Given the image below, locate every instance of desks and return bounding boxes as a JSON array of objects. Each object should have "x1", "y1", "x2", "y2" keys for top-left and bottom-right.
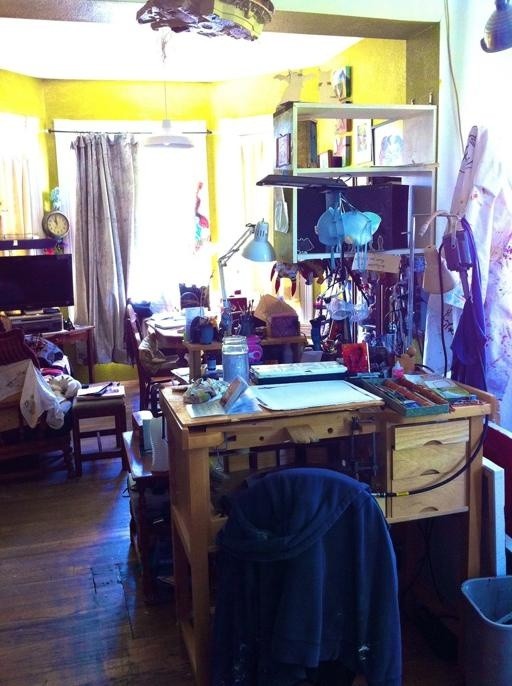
[
  {"x1": 0, "y1": 325, "x2": 94, "y2": 386},
  {"x1": 159, "y1": 371, "x2": 497, "y2": 686}
]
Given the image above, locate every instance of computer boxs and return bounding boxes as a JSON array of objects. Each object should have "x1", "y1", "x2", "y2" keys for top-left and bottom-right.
[{"x1": 8, "y1": 312, "x2": 64, "y2": 335}]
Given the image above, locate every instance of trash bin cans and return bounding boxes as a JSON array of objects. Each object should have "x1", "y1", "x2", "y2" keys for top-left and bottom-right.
[{"x1": 460, "y1": 576, "x2": 511, "y2": 686}]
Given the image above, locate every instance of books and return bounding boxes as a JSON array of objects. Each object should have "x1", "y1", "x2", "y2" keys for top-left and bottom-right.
[{"x1": 184, "y1": 375, "x2": 263, "y2": 417}]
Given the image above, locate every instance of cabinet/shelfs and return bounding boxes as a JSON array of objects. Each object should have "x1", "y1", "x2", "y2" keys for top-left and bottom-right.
[{"x1": 272, "y1": 105, "x2": 435, "y2": 263}]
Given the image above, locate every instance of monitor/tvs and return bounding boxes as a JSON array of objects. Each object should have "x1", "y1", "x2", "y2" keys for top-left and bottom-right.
[{"x1": 0, "y1": 252, "x2": 73, "y2": 313}]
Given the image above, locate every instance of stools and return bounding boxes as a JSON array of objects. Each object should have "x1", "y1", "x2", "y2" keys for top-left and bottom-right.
[{"x1": 72, "y1": 383, "x2": 129, "y2": 473}]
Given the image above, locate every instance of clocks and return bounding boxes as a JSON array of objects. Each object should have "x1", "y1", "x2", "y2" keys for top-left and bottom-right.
[{"x1": 43, "y1": 211, "x2": 70, "y2": 239}]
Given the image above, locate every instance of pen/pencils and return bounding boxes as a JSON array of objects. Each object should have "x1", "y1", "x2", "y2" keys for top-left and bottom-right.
[{"x1": 236, "y1": 299, "x2": 254, "y2": 315}]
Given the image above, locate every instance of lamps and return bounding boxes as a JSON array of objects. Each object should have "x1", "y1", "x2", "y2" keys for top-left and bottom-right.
[
  {"x1": 144, "y1": 28, "x2": 194, "y2": 148},
  {"x1": 218, "y1": 218, "x2": 276, "y2": 308},
  {"x1": 477, "y1": 0, "x2": 512, "y2": 54}
]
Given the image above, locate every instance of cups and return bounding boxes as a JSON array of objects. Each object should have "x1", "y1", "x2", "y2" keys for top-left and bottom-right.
[{"x1": 222, "y1": 336, "x2": 249, "y2": 383}]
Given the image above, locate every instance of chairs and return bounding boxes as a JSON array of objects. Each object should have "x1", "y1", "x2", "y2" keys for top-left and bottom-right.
[
  {"x1": 0, "y1": 398, "x2": 73, "y2": 468},
  {"x1": 127, "y1": 305, "x2": 176, "y2": 410}
]
